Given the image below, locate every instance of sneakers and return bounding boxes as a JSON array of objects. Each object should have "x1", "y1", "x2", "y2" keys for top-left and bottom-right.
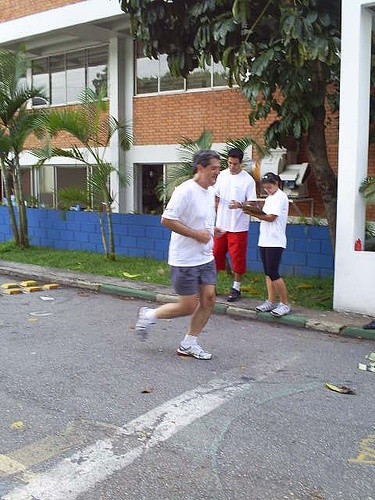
[
  {"x1": 176, "y1": 341, "x2": 212, "y2": 360},
  {"x1": 135, "y1": 306, "x2": 155, "y2": 341},
  {"x1": 256, "y1": 299, "x2": 276, "y2": 312},
  {"x1": 227, "y1": 288, "x2": 242, "y2": 302},
  {"x1": 271, "y1": 301, "x2": 290, "y2": 317}
]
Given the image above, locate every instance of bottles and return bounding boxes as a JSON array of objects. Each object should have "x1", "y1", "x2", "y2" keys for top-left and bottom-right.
[{"x1": 356, "y1": 238, "x2": 362, "y2": 251}]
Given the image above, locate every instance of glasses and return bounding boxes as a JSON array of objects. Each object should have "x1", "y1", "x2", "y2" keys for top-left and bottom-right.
[{"x1": 263, "y1": 175, "x2": 273, "y2": 180}]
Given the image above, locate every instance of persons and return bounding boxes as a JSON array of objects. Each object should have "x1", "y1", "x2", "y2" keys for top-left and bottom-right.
[
  {"x1": 211, "y1": 148, "x2": 258, "y2": 303},
  {"x1": 244, "y1": 172, "x2": 292, "y2": 317},
  {"x1": 136, "y1": 150, "x2": 225, "y2": 360}
]
